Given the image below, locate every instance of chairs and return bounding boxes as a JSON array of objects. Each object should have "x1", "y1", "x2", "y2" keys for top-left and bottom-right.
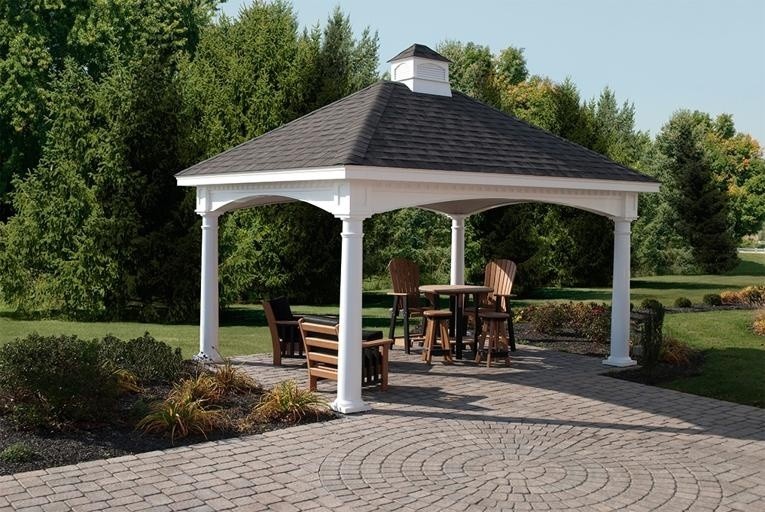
[
  {"x1": 464, "y1": 257, "x2": 518, "y2": 358},
  {"x1": 297, "y1": 314, "x2": 393, "y2": 392},
  {"x1": 261, "y1": 296, "x2": 304, "y2": 366},
  {"x1": 387, "y1": 256, "x2": 440, "y2": 355}
]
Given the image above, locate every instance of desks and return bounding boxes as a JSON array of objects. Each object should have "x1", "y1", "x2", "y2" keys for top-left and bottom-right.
[{"x1": 418, "y1": 284, "x2": 494, "y2": 359}]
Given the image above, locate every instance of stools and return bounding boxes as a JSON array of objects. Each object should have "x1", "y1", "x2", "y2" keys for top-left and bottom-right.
[
  {"x1": 475, "y1": 312, "x2": 512, "y2": 368},
  {"x1": 422, "y1": 310, "x2": 453, "y2": 366}
]
